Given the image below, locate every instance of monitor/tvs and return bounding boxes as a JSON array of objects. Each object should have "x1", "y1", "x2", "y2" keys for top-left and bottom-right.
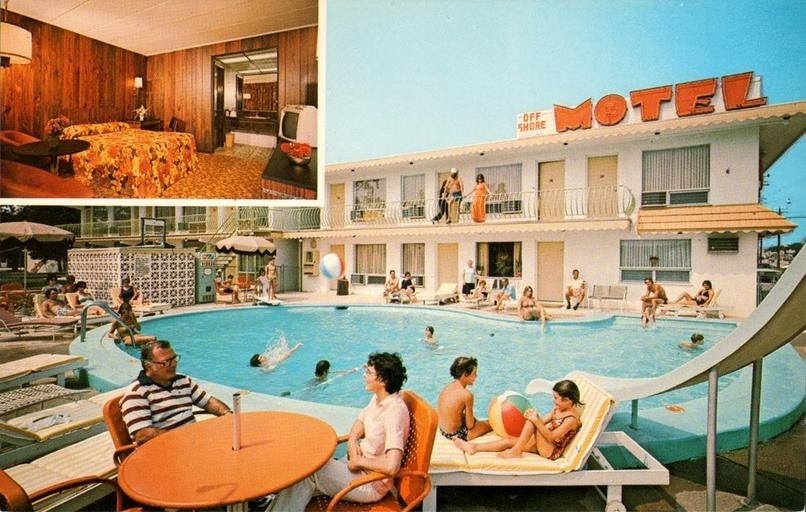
[{"x1": 278, "y1": 105, "x2": 317, "y2": 148}]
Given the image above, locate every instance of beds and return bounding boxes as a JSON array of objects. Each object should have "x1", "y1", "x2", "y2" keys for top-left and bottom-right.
[
  {"x1": 60, "y1": 122, "x2": 199, "y2": 199},
  {"x1": 12, "y1": 140, "x2": 90, "y2": 175}
]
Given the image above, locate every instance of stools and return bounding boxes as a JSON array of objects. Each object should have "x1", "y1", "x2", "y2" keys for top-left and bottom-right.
[{"x1": 569, "y1": 296, "x2": 585, "y2": 309}]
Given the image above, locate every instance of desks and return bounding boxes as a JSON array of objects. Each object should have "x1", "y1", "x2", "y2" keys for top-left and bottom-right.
[
  {"x1": 262, "y1": 140, "x2": 316, "y2": 199},
  {"x1": 238, "y1": 288, "x2": 256, "y2": 300},
  {"x1": 118, "y1": 411, "x2": 338, "y2": 510}
]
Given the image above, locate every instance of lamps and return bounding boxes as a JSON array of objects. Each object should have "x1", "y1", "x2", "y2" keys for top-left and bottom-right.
[{"x1": 0, "y1": 0, "x2": 32, "y2": 66}]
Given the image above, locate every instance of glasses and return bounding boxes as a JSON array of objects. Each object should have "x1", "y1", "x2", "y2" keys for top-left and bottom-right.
[{"x1": 150, "y1": 355, "x2": 180, "y2": 368}]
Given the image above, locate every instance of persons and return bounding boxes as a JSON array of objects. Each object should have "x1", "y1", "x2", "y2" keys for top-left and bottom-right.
[
  {"x1": 431, "y1": 168, "x2": 493, "y2": 224},
  {"x1": 400, "y1": 272, "x2": 416, "y2": 304},
  {"x1": 640, "y1": 277, "x2": 714, "y2": 330},
  {"x1": 117, "y1": 341, "x2": 277, "y2": 511},
  {"x1": 463, "y1": 260, "x2": 554, "y2": 320},
  {"x1": 565, "y1": 270, "x2": 587, "y2": 311},
  {"x1": 452, "y1": 380, "x2": 585, "y2": 460},
  {"x1": 424, "y1": 326, "x2": 438, "y2": 344},
  {"x1": 40, "y1": 275, "x2": 157, "y2": 346},
  {"x1": 385, "y1": 270, "x2": 401, "y2": 303},
  {"x1": 314, "y1": 360, "x2": 331, "y2": 379},
  {"x1": 215, "y1": 257, "x2": 279, "y2": 306},
  {"x1": 680, "y1": 333, "x2": 705, "y2": 349},
  {"x1": 264, "y1": 352, "x2": 411, "y2": 512},
  {"x1": 486, "y1": 391, "x2": 534, "y2": 440},
  {"x1": 437, "y1": 357, "x2": 493, "y2": 440},
  {"x1": 250, "y1": 337, "x2": 303, "y2": 373}
]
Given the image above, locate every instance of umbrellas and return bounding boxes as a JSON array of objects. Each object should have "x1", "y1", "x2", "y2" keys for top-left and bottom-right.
[
  {"x1": 0, "y1": 221, "x2": 73, "y2": 296},
  {"x1": 214, "y1": 236, "x2": 278, "y2": 302}
]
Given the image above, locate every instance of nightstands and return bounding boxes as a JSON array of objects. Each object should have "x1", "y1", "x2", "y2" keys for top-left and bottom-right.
[{"x1": 127, "y1": 120, "x2": 162, "y2": 131}]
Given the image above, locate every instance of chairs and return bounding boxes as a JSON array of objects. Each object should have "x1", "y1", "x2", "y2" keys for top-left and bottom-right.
[
  {"x1": 422, "y1": 375, "x2": 670, "y2": 512},
  {"x1": 305, "y1": 391, "x2": 437, "y2": 512},
  {"x1": 0, "y1": 288, "x2": 172, "y2": 512},
  {"x1": 386, "y1": 287, "x2": 436, "y2": 304},
  {"x1": 500, "y1": 280, "x2": 523, "y2": 310},
  {"x1": 0, "y1": 129, "x2": 40, "y2": 146},
  {"x1": 655, "y1": 289, "x2": 722, "y2": 317},
  {"x1": 0, "y1": 158, "x2": 94, "y2": 198},
  {"x1": 213, "y1": 279, "x2": 233, "y2": 305},
  {"x1": 416, "y1": 283, "x2": 460, "y2": 305},
  {"x1": 586, "y1": 284, "x2": 628, "y2": 312},
  {"x1": 465, "y1": 278, "x2": 497, "y2": 309}
]
